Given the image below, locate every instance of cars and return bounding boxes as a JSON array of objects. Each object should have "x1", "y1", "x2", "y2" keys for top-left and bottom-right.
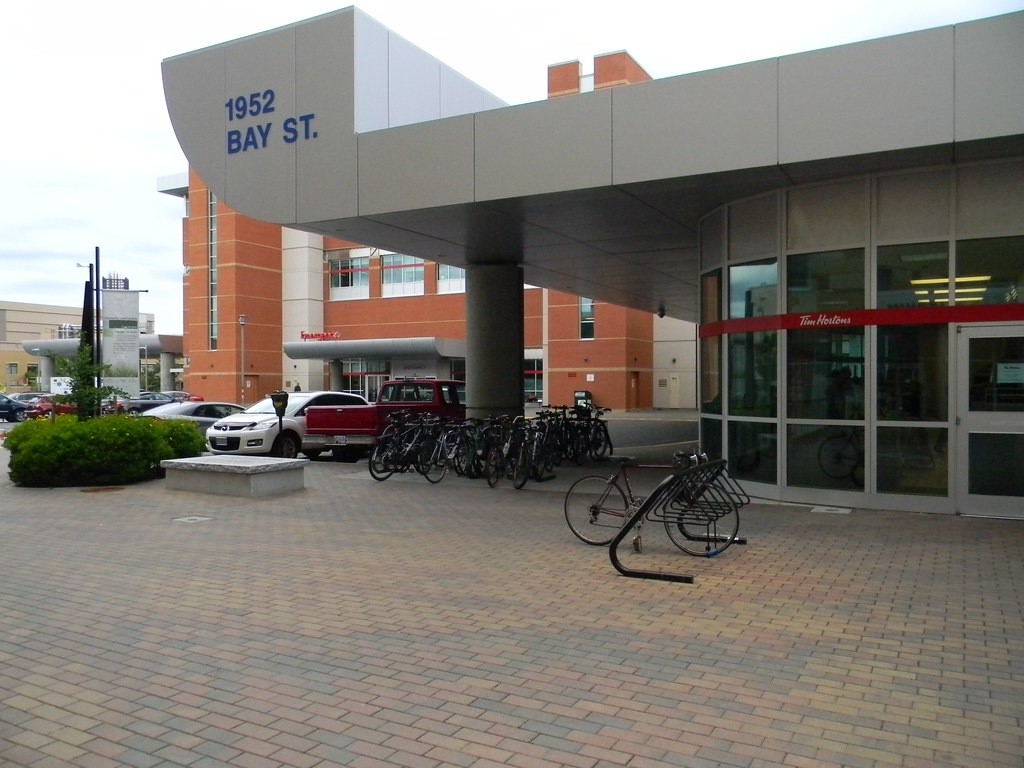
[
  {"x1": 0, "y1": 391, "x2": 204, "y2": 421},
  {"x1": 129, "y1": 401, "x2": 246, "y2": 444},
  {"x1": 204, "y1": 391, "x2": 374, "y2": 460}
]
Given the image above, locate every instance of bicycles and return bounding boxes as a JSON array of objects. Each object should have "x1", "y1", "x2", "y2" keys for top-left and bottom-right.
[
  {"x1": 816, "y1": 404, "x2": 933, "y2": 477},
  {"x1": 564, "y1": 452, "x2": 739, "y2": 557},
  {"x1": 369, "y1": 402, "x2": 613, "y2": 490}
]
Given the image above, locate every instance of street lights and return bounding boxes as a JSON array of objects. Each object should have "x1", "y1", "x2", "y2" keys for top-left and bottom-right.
[{"x1": 238, "y1": 314, "x2": 248, "y2": 406}]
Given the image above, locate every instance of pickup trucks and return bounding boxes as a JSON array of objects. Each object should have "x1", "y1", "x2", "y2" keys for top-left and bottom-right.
[{"x1": 302, "y1": 379, "x2": 465, "y2": 462}]
